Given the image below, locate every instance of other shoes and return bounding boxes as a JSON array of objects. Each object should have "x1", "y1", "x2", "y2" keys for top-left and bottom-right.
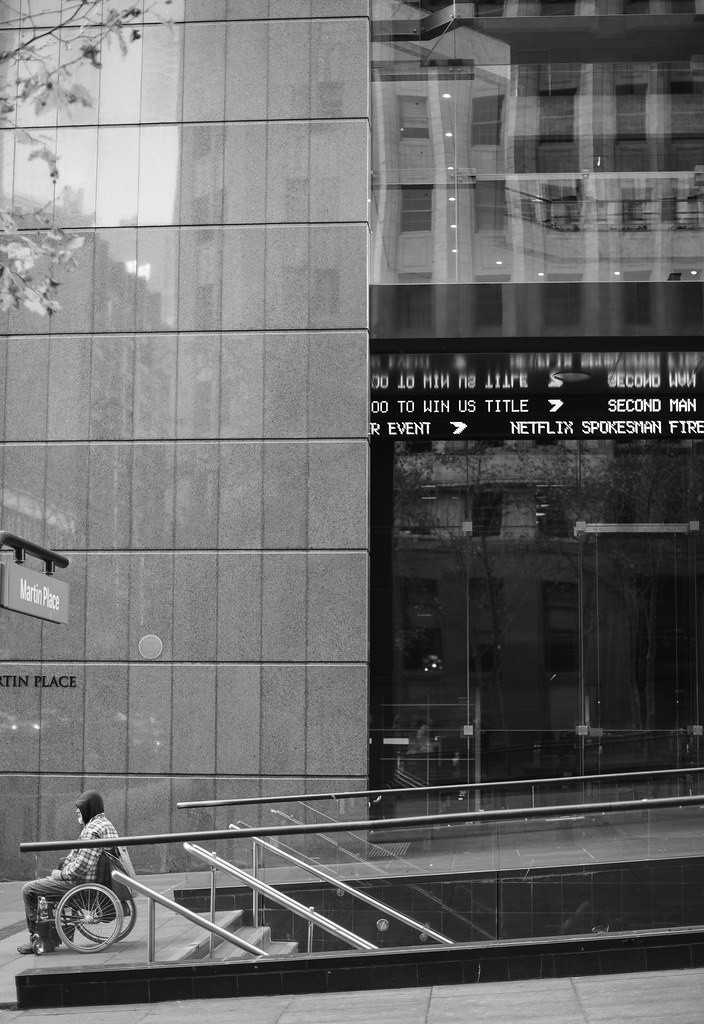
[{"x1": 18, "y1": 943, "x2": 55, "y2": 954}]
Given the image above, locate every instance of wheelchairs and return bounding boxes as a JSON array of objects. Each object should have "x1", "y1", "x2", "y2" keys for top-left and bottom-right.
[{"x1": 31, "y1": 857, "x2": 137, "y2": 955}]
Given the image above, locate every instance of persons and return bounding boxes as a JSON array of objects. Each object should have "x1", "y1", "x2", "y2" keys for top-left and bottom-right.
[{"x1": 17, "y1": 788, "x2": 118, "y2": 955}]
